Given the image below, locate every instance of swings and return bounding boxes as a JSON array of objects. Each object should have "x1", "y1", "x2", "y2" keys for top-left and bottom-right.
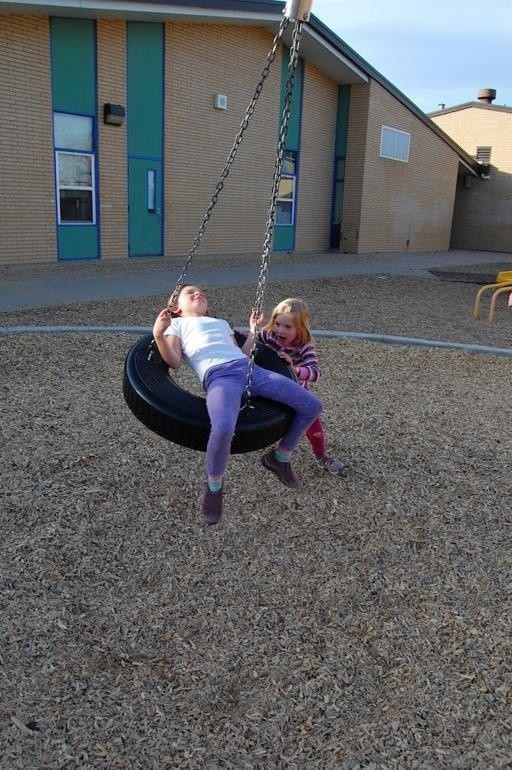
[{"x1": 122, "y1": 1, "x2": 312, "y2": 452}]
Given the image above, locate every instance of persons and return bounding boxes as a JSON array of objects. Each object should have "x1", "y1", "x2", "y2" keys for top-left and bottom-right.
[
  {"x1": 152, "y1": 284, "x2": 323, "y2": 525},
  {"x1": 238, "y1": 298, "x2": 345, "y2": 475}
]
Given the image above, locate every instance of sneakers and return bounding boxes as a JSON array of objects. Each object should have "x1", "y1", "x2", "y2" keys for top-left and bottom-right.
[
  {"x1": 260, "y1": 450, "x2": 299, "y2": 489},
  {"x1": 202, "y1": 482, "x2": 223, "y2": 524},
  {"x1": 315, "y1": 454, "x2": 346, "y2": 476}
]
